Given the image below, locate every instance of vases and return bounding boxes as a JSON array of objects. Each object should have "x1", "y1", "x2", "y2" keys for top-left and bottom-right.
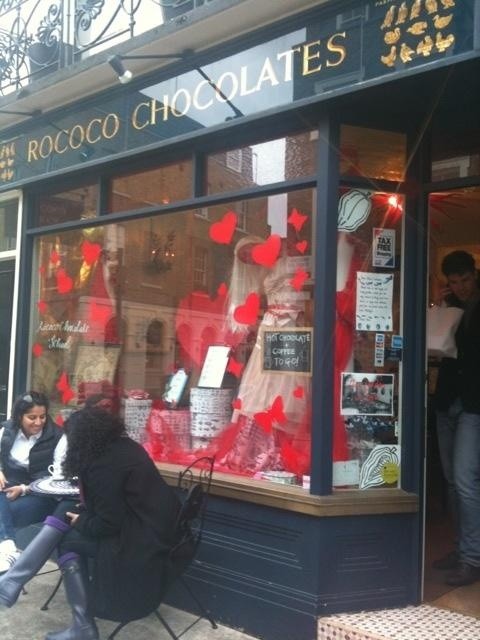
[{"x1": 28, "y1": 42, "x2": 81, "y2": 82}]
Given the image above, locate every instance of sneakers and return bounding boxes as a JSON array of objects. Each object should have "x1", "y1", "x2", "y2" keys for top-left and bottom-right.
[
  {"x1": 446, "y1": 565, "x2": 479, "y2": 587},
  {"x1": 434, "y1": 551, "x2": 460, "y2": 570}
]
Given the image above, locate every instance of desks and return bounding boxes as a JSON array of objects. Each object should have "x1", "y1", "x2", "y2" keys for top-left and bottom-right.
[{"x1": 28, "y1": 477, "x2": 80, "y2": 610}]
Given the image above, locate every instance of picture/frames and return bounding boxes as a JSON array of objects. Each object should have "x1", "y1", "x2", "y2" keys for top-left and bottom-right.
[{"x1": 259, "y1": 327, "x2": 313, "y2": 377}]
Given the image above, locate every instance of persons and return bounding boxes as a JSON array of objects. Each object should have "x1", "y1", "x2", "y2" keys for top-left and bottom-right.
[
  {"x1": 0, "y1": 407, "x2": 195, "y2": 639},
  {"x1": 223, "y1": 235, "x2": 306, "y2": 433},
  {"x1": 431, "y1": 249, "x2": 480, "y2": 586},
  {"x1": 332, "y1": 232, "x2": 363, "y2": 458},
  {"x1": 1, "y1": 392, "x2": 70, "y2": 573}
]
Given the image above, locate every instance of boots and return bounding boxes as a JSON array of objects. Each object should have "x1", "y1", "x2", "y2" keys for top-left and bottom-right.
[
  {"x1": 0, "y1": 515, "x2": 72, "y2": 609},
  {"x1": 45, "y1": 552, "x2": 103, "y2": 640}
]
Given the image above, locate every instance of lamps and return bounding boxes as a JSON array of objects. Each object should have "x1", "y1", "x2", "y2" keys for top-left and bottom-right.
[{"x1": 108, "y1": 50, "x2": 195, "y2": 85}]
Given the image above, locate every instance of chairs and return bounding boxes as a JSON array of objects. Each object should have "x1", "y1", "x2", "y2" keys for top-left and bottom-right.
[{"x1": 106, "y1": 456, "x2": 216, "y2": 640}]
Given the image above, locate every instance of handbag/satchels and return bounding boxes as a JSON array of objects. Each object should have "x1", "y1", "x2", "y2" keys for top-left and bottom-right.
[{"x1": 426, "y1": 301, "x2": 464, "y2": 361}]
